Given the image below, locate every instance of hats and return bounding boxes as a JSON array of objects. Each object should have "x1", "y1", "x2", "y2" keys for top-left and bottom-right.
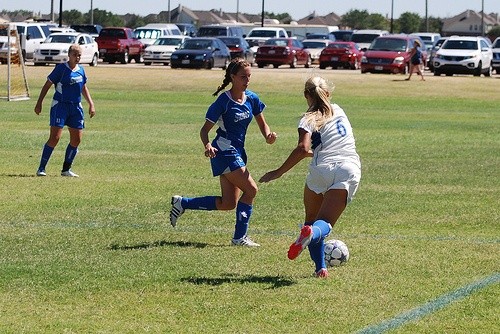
[{"x1": 414, "y1": 39, "x2": 422, "y2": 48}]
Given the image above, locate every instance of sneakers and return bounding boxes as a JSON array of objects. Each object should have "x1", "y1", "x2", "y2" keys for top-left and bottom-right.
[
  {"x1": 170, "y1": 195, "x2": 185, "y2": 228},
  {"x1": 314, "y1": 267, "x2": 329, "y2": 280},
  {"x1": 61, "y1": 170, "x2": 79, "y2": 178},
  {"x1": 288, "y1": 225, "x2": 313, "y2": 260},
  {"x1": 230, "y1": 235, "x2": 260, "y2": 247},
  {"x1": 37, "y1": 171, "x2": 46, "y2": 176}
]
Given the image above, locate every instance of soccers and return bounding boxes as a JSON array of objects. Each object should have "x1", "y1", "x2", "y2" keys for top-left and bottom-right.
[{"x1": 323, "y1": 240, "x2": 349, "y2": 267}]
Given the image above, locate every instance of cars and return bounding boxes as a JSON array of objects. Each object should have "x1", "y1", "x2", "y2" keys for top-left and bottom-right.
[
  {"x1": 360, "y1": 36, "x2": 426, "y2": 75},
  {"x1": 217, "y1": 37, "x2": 253, "y2": 66},
  {"x1": 433, "y1": 35, "x2": 493, "y2": 77},
  {"x1": 319, "y1": 41, "x2": 365, "y2": 69},
  {"x1": 33, "y1": 32, "x2": 99, "y2": 67},
  {"x1": 254, "y1": 38, "x2": 312, "y2": 68},
  {"x1": 170, "y1": 37, "x2": 231, "y2": 71},
  {"x1": 492, "y1": 37, "x2": 500, "y2": 75},
  {"x1": 142, "y1": 36, "x2": 192, "y2": 65},
  {"x1": 49, "y1": 22, "x2": 448, "y2": 58}
]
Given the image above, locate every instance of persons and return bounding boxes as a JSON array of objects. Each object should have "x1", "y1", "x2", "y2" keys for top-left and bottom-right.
[
  {"x1": 32, "y1": 45, "x2": 95, "y2": 178},
  {"x1": 405, "y1": 40, "x2": 427, "y2": 80},
  {"x1": 259, "y1": 77, "x2": 362, "y2": 277},
  {"x1": 169, "y1": 58, "x2": 277, "y2": 247}
]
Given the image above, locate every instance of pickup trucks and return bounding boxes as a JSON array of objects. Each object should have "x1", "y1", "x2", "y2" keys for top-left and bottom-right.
[{"x1": 94, "y1": 27, "x2": 143, "y2": 64}]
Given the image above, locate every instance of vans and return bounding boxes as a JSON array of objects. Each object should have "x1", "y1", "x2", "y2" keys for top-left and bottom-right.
[{"x1": 0, "y1": 22, "x2": 46, "y2": 64}]
